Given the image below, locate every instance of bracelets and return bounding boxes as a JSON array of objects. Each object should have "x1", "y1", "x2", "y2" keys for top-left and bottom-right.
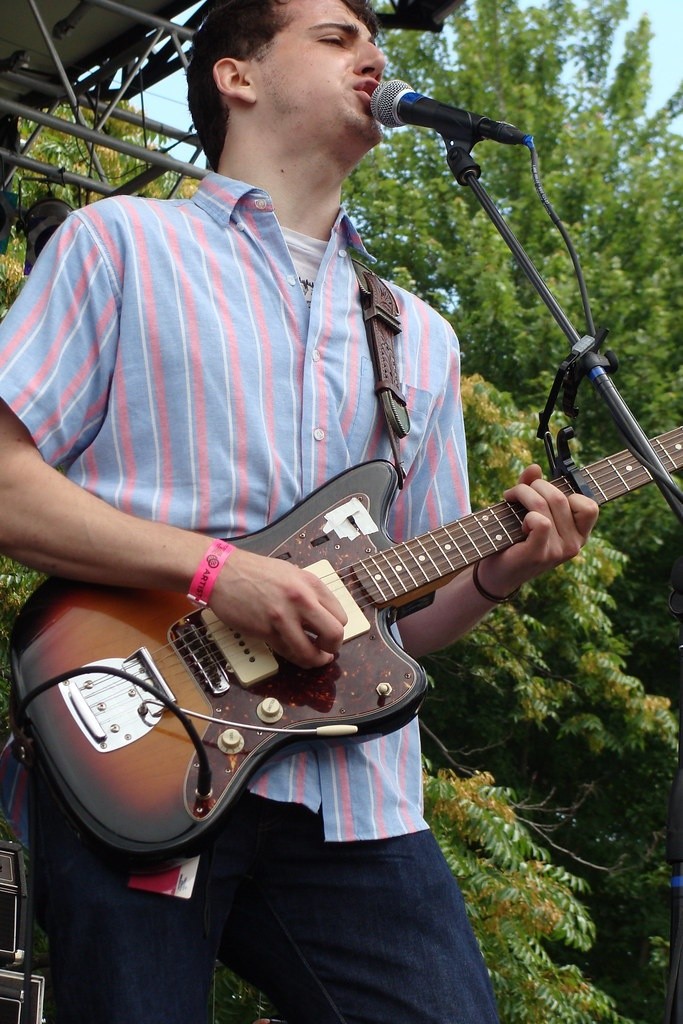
[
  {"x1": 186, "y1": 539, "x2": 236, "y2": 609},
  {"x1": 473, "y1": 560, "x2": 521, "y2": 603}
]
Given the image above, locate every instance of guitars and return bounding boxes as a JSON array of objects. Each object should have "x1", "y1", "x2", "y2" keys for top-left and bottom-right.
[{"x1": 8, "y1": 421, "x2": 681, "y2": 864}]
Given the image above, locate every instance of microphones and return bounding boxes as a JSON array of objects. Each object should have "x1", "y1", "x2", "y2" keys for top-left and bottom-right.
[{"x1": 370, "y1": 80, "x2": 530, "y2": 146}]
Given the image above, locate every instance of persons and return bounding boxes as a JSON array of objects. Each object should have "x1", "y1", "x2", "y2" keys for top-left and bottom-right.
[{"x1": 1, "y1": 0, "x2": 598, "y2": 1023}]
